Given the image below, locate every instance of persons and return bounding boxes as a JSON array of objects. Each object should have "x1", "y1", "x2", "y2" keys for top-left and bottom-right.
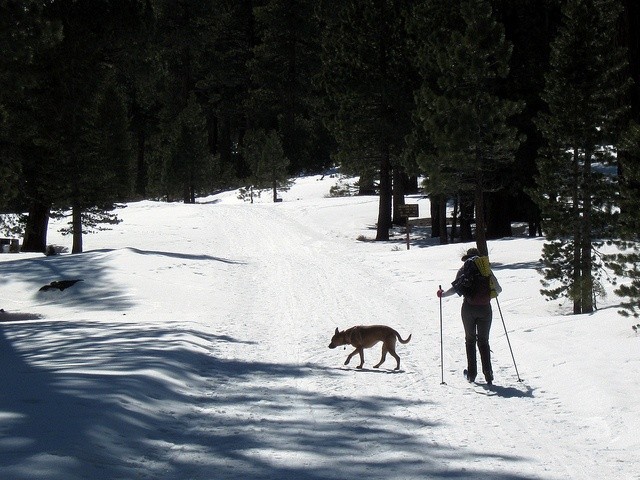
[{"x1": 437, "y1": 248, "x2": 502, "y2": 384}]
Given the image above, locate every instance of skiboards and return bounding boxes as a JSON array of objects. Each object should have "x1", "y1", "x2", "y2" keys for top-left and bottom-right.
[{"x1": 462, "y1": 368, "x2": 494, "y2": 390}]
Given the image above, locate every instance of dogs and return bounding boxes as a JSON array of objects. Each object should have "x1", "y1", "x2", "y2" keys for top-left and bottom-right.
[{"x1": 328, "y1": 325, "x2": 412, "y2": 371}]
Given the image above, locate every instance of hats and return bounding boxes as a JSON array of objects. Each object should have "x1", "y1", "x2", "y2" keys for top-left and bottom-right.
[{"x1": 462, "y1": 248, "x2": 482, "y2": 262}]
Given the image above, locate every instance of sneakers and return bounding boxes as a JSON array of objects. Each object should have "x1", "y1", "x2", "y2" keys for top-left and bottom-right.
[
  {"x1": 484, "y1": 370, "x2": 493, "y2": 382},
  {"x1": 466, "y1": 372, "x2": 476, "y2": 381}
]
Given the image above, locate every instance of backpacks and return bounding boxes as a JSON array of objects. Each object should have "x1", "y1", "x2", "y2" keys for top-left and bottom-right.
[{"x1": 464, "y1": 256, "x2": 492, "y2": 300}]
